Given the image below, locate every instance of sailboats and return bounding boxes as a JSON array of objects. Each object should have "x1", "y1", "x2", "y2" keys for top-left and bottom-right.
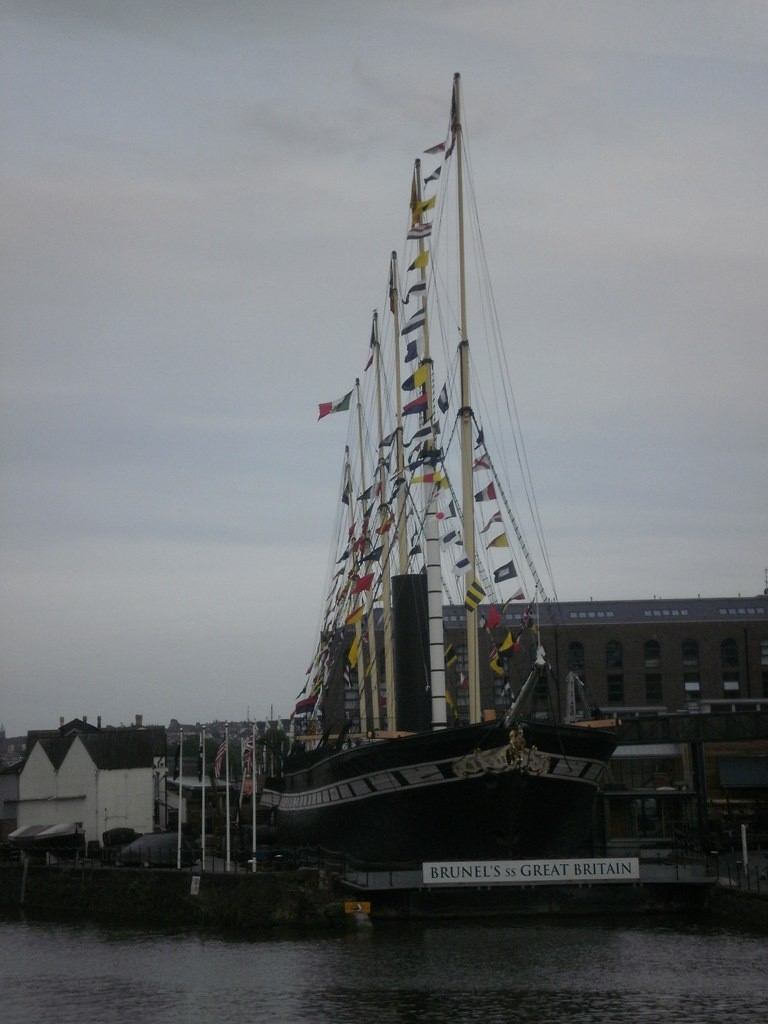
[{"x1": 238, "y1": 70, "x2": 619, "y2": 871}]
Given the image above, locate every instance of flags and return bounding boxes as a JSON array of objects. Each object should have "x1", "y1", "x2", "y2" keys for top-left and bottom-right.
[
  {"x1": 243, "y1": 730, "x2": 253, "y2": 773},
  {"x1": 173, "y1": 737, "x2": 179, "y2": 780},
  {"x1": 215, "y1": 732, "x2": 226, "y2": 778},
  {"x1": 197, "y1": 731, "x2": 203, "y2": 782},
  {"x1": 407, "y1": 222, "x2": 431, "y2": 239},
  {"x1": 412, "y1": 194, "x2": 436, "y2": 228},
  {"x1": 402, "y1": 392, "x2": 428, "y2": 417},
  {"x1": 401, "y1": 309, "x2": 425, "y2": 335},
  {"x1": 405, "y1": 336, "x2": 425, "y2": 363},
  {"x1": 401, "y1": 282, "x2": 427, "y2": 305},
  {"x1": 289, "y1": 420, "x2": 539, "y2": 722},
  {"x1": 365, "y1": 325, "x2": 377, "y2": 371},
  {"x1": 438, "y1": 384, "x2": 449, "y2": 414},
  {"x1": 424, "y1": 166, "x2": 441, "y2": 189},
  {"x1": 423, "y1": 141, "x2": 445, "y2": 154},
  {"x1": 317, "y1": 388, "x2": 353, "y2": 421},
  {"x1": 401, "y1": 364, "x2": 426, "y2": 391},
  {"x1": 407, "y1": 251, "x2": 428, "y2": 272}
]
